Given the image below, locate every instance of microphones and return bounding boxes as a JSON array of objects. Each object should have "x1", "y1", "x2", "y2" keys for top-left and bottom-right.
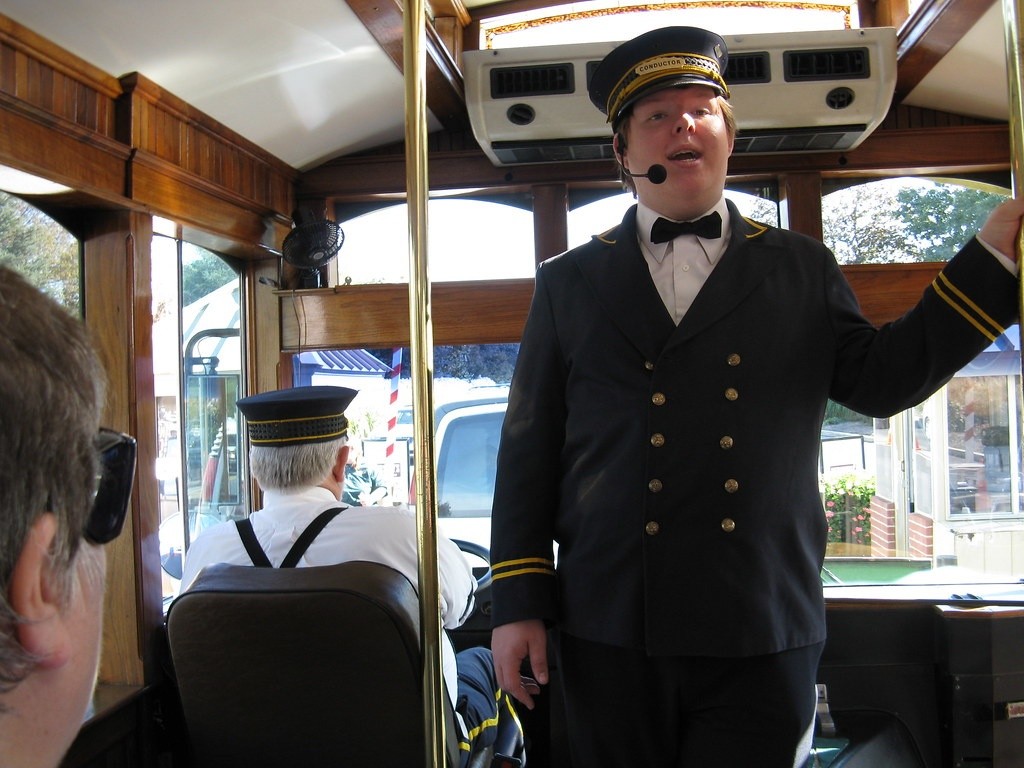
[{"x1": 621, "y1": 152, "x2": 668, "y2": 184}]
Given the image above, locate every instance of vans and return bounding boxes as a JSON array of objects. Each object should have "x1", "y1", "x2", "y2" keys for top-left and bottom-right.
[{"x1": 405, "y1": 393, "x2": 559, "y2": 571}]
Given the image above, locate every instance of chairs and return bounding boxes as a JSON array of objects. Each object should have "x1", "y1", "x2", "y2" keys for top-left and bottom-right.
[{"x1": 167, "y1": 559, "x2": 498, "y2": 767}]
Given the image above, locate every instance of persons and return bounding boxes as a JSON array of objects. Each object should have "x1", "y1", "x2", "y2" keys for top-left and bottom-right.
[
  {"x1": 490, "y1": 26, "x2": 1024, "y2": 768},
  {"x1": 0, "y1": 260, "x2": 137, "y2": 768},
  {"x1": 179, "y1": 386, "x2": 526, "y2": 768}
]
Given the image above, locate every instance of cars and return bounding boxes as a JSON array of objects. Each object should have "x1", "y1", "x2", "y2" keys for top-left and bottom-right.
[{"x1": 380, "y1": 408, "x2": 414, "y2": 465}]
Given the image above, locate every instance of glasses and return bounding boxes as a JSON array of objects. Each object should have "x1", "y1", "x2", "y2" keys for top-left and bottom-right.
[{"x1": 50, "y1": 427, "x2": 138, "y2": 544}]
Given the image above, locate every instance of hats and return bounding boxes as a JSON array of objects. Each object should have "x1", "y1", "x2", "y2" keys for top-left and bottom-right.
[
  {"x1": 237, "y1": 385, "x2": 358, "y2": 447},
  {"x1": 587, "y1": 26, "x2": 728, "y2": 131}
]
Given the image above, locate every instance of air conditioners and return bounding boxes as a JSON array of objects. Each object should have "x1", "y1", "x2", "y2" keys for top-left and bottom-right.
[{"x1": 461, "y1": 25, "x2": 897, "y2": 170}]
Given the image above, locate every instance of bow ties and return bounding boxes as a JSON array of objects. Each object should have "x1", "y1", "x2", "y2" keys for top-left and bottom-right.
[{"x1": 650, "y1": 210, "x2": 722, "y2": 243}]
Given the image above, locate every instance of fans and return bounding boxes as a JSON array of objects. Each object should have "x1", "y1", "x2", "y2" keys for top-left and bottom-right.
[{"x1": 280, "y1": 205, "x2": 345, "y2": 270}]
